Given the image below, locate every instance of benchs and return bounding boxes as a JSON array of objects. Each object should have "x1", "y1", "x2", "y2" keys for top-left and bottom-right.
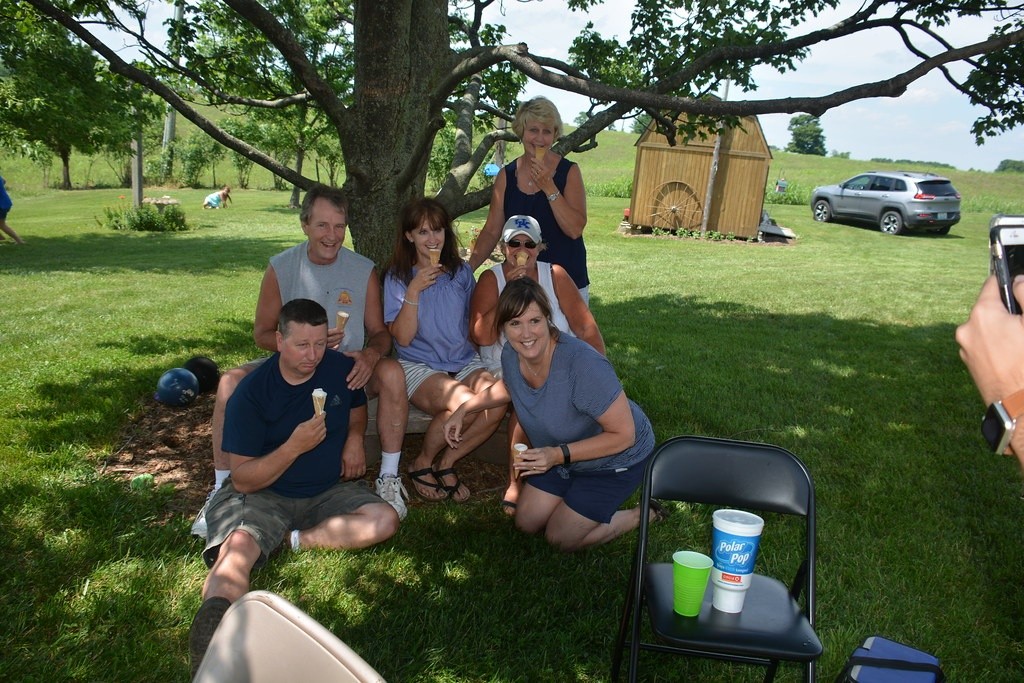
[{"x1": 362, "y1": 395, "x2": 513, "y2": 469}]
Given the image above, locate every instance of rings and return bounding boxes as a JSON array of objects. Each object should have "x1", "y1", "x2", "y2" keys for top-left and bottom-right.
[{"x1": 534, "y1": 169, "x2": 541, "y2": 179}]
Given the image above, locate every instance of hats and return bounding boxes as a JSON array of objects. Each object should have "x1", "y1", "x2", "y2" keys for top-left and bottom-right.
[{"x1": 501, "y1": 215, "x2": 542, "y2": 244}]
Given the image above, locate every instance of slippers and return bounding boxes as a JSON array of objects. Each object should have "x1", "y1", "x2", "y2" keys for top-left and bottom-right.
[
  {"x1": 504, "y1": 501, "x2": 517, "y2": 517},
  {"x1": 432, "y1": 464, "x2": 469, "y2": 503},
  {"x1": 641, "y1": 494, "x2": 670, "y2": 525},
  {"x1": 408, "y1": 468, "x2": 448, "y2": 502}
]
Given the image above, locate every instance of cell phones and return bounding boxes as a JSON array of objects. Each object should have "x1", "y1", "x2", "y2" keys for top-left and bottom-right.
[{"x1": 988, "y1": 214, "x2": 1024, "y2": 316}]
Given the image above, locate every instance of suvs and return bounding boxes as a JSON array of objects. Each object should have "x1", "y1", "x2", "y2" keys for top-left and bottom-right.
[{"x1": 809, "y1": 170, "x2": 962, "y2": 236}]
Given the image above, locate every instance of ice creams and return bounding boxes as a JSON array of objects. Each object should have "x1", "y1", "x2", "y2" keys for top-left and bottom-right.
[
  {"x1": 430, "y1": 249, "x2": 441, "y2": 265},
  {"x1": 535, "y1": 145, "x2": 546, "y2": 162},
  {"x1": 516, "y1": 251, "x2": 528, "y2": 267},
  {"x1": 514, "y1": 444, "x2": 528, "y2": 478},
  {"x1": 336, "y1": 311, "x2": 350, "y2": 330},
  {"x1": 312, "y1": 388, "x2": 327, "y2": 416}
]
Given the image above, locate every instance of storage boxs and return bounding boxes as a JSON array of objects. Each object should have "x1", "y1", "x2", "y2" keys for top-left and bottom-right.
[{"x1": 835, "y1": 636, "x2": 947, "y2": 683}]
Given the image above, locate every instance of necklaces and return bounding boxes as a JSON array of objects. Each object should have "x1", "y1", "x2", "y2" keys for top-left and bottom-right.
[
  {"x1": 528, "y1": 180, "x2": 533, "y2": 187},
  {"x1": 524, "y1": 360, "x2": 542, "y2": 376}
]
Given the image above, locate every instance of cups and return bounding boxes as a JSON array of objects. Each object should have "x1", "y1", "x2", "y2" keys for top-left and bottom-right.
[
  {"x1": 673, "y1": 550, "x2": 714, "y2": 616},
  {"x1": 710, "y1": 509, "x2": 764, "y2": 613}
]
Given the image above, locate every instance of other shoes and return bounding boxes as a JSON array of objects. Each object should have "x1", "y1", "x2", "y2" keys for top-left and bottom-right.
[{"x1": 187, "y1": 596, "x2": 231, "y2": 676}]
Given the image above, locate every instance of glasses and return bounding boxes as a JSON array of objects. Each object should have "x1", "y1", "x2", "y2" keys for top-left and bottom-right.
[{"x1": 508, "y1": 239, "x2": 539, "y2": 249}]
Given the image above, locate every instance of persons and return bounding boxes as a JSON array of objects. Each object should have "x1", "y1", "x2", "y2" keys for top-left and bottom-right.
[
  {"x1": 189, "y1": 298, "x2": 399, "y2": 681},
  {"x1": 496, "y1": 277, "x2": 674, "y2": 549},
  {"x1": 190, "y1": 184, "x2": 408, "y2": 539},
  {"x1": 203, "y1": 187, "x2": 231, "y2": 210},
  {"x1": 383, "y1": 199, "x2": 507, "y2": 500},
  {"x1": 0, "y1": 176, "x2": 25, "y2": 245},
  {"x1": 468, "y1": 215, "x2": 608, "y2": 519},
  {"x1": 955, "y1": 269, "x2": 1024, "y2": 471},
  {"x1": 469, "y1": 98, "x2": 590, "y2": 316}
]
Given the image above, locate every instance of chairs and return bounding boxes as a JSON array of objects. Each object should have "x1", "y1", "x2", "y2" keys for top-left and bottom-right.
[
  {"x1": 192, "y1": 590, "x2": 386, "y2": 683},
  {"x1": 606, "y1": 436, "x2": 823, "y2": 683}
]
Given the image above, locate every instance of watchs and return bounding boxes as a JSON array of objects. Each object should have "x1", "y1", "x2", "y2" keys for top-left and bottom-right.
[
  {"x1": 546, "y1": 191, "x2": 560, "y2": 202},
  {"x1": 980, "y1": 389, "x2": 1024, "y2": 455}
]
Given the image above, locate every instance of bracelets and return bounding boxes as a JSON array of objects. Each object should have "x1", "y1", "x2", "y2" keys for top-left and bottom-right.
[
  {"x1": 559, "y1": 443, "x2": 571, "y2": 468},
  {"x1": 404, "y1": 298, "x2": 419, "y2": 308}
]
